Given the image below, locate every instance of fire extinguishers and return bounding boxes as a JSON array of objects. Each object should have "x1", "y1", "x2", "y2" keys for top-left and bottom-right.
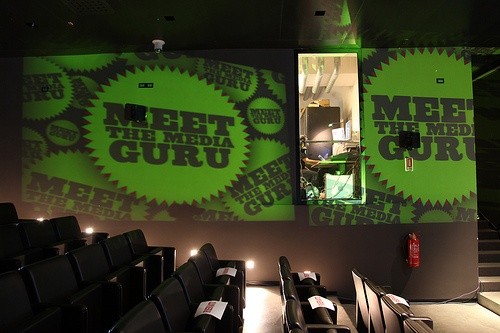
[{"x1": 408, "y1": 233, "x2": 421, "y2": 268}]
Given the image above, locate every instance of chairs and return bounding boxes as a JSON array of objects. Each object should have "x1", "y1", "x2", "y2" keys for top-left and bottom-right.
[
  {"x1": 0, "y1": 204, "x2": 247, "y2": 333},
  {"x1": 278, "y1": 256, "x2": 434, "y2": 333}
]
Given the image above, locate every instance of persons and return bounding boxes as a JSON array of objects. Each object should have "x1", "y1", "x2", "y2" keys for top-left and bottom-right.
[{"x1": 300, "y1": 135, "x2": 332, "y2": 189}]
